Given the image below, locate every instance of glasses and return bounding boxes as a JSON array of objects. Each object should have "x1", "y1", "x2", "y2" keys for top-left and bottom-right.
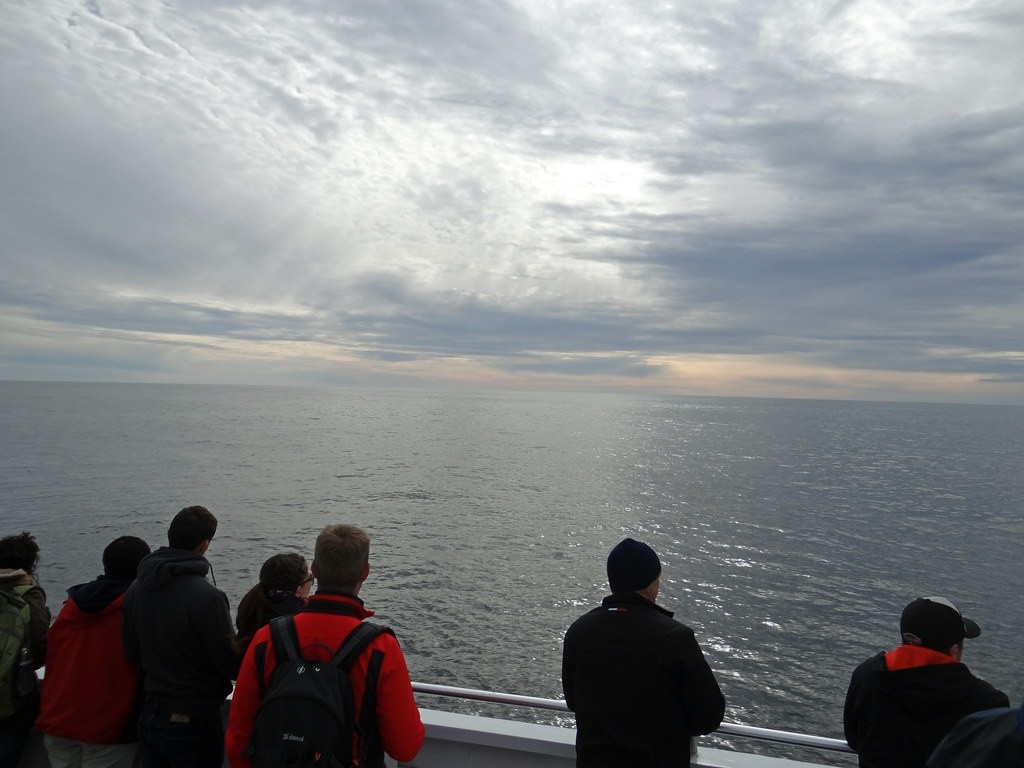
[{"x1": 296, "y1": 573, "x2": 314, "y2": 589}]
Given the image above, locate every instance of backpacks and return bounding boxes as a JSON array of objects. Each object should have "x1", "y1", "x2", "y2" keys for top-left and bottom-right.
[
  {"x1": 0, "y1": 584, "x2": 35, "y2": 719},
  {"x1": 241, "y1": 614, "x2": 400, "y2": 768}
]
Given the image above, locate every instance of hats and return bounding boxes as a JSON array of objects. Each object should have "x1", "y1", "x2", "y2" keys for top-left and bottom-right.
[
  {"x1": 900, "y1": 597, "x2": 981, "y2": 647},
  {"x1": 607, "y1": 538, "x2": 661, "y2": 593}
]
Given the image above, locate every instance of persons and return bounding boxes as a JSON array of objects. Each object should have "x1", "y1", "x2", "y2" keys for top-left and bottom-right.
[
  {"x1": 226, "y1": 549, "x2": 313, "y2": 680},
  {"x1": 562, "y1": 538, "x2": 727, "y2": 768},
  {"x1": 119, "y1": 504, "x2": 238, "y2": 768},
  {"x1": 925, "y1": 704, "x2": 1024, "y2": 768},
  {"x1": 40, "y1": 535, "x2": 151, "y2": 768},
  {"x1": 225, "y1": 522, "x2": 426, "y2": 768},
  {"x1": 0, "y1": 530, "x2": 52, "y2": 768},
  {"x1": 843, "y1": 596, "x2": 1010, "y2": 768}
]
